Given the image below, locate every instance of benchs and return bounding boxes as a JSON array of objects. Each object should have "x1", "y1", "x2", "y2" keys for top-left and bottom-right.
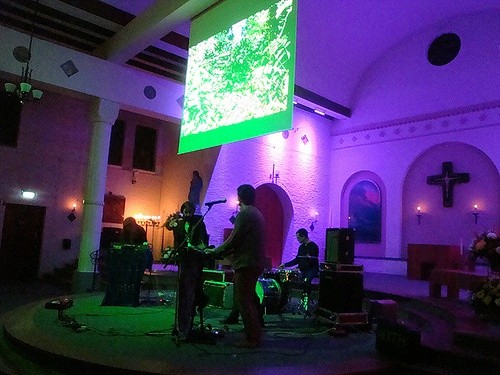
[{"x1": 430, "y1": 268, "x2": 492, "y2": 301}]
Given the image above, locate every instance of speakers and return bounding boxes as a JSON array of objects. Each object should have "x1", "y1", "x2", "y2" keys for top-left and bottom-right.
[
  {"x1": 98, "y1": 227, "x2": 123, "y2": 271},
  {"x1": 375, "y1": 318, "x2": 422, "y2": 363},
  {"x1": 325, "y1": 228, "x2": 354, "y2": 264},
  {"x1": 203, "y1": 280, "x2": 235, "y2": 309},
  {"x1": 318, "y1": 270, "x2": 363, "y2": 314}
]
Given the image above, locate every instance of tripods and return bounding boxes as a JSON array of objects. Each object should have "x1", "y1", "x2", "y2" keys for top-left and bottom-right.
[{"x1": 145, "y1": 206, "x2": 213, "y2": 346}]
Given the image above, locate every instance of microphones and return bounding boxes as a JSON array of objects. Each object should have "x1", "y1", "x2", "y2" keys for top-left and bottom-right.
[{"x1": 205, "y1": 199, "x2": 227, "y2": 206}]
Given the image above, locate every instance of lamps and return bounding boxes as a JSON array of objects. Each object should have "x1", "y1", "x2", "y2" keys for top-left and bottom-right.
[{"x1": 5, "y1": 0, "x2": 43, "y2": 105}]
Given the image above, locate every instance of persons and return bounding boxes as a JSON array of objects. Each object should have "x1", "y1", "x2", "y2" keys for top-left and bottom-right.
[
  {"x1": 203, "y1": 185, "x2": 267, "y2": 347},
  {"x1": 166, "y1": 201, "x2": 209, "y2": 315},
  {"x1": 120, "y1": 218, "x2": 148, "y2": 246},
  {"x1": 279, "y1": 228, "x2": 319, "y2": 292}
]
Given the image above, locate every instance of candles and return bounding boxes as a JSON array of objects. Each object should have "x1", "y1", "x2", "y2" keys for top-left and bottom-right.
[
  {"x1": 460, "y1": 237, "x2": 463, "y2": 256},
  {"x1": 474, "y1": 204, "x2": 477, "y2": 212},
  {"x1": 418, "y1": 207, "x2": 421, "y2": 214}
]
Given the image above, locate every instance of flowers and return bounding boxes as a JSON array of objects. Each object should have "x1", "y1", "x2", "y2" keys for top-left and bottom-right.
[
  {"x1": 472, "y1": 230, "x2": 500, "y2": 270},
  {"x1": 463, "y1": 272, "x2": 500, "y2": 320}
]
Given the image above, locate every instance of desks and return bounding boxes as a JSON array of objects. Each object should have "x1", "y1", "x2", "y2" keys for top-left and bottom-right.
[{"x1": 407, "y1": 244, "x2": 459, "y2": 280}]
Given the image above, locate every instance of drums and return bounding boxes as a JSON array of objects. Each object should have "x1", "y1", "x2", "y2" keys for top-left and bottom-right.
[
  {"x1": 263, "y1": 271, "x2": 302, "y2": 286},
  {"x1": 254, "y1": 278, "x2": 282, "y2": 308}
]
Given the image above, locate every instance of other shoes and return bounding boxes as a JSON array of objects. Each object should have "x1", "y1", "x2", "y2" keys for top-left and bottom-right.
[{"x1": 219, "y1": 317, "x2": 239, "y2": 324}]
